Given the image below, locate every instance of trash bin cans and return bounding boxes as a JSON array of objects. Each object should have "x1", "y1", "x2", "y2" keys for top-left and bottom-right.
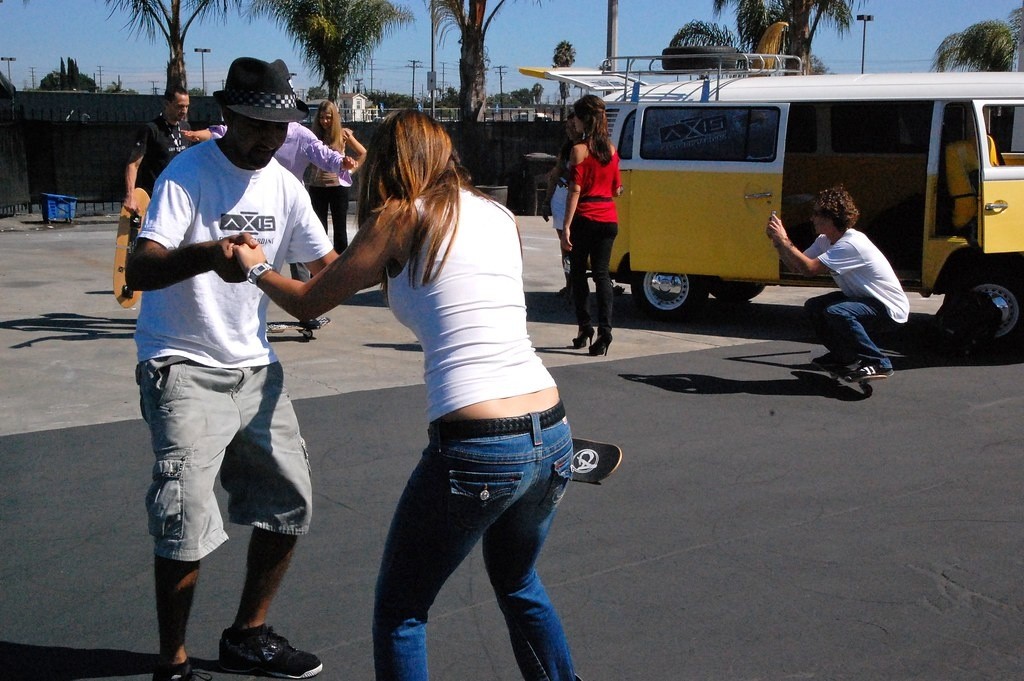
[{"x1": 38, "y1": 192, "x2": 77, "y2": 224}]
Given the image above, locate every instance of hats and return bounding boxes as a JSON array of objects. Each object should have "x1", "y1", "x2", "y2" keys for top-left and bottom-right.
[{"x1": 214, "y1": 51, "x2": 308, "y2": 124}]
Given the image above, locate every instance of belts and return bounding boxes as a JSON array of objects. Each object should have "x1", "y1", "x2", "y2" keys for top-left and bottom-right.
[{"x1": 428, "y1": 399, "x2": 565, "y2": 440}]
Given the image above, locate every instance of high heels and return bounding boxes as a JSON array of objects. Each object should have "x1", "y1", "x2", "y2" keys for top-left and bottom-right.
[
  {"x1": 589, "y1": 332, "x2": 612, "y2": 357},
  {"x1": 572, "y1": 325, "x2": 595, "y2": 349}
]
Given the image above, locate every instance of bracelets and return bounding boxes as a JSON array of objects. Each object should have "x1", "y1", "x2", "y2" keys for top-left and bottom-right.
[{"x1": 245, "y1": 263, "x2": 261, "y2": 285}]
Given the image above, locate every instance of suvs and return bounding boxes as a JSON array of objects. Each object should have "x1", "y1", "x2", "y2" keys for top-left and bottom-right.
[{"x1": 300, "y1": 108, "x2": 319, "y2": 123}]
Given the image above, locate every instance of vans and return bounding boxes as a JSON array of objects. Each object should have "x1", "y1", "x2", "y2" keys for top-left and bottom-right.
[{"x1": 517, "y1": 52, "x2": 1024, "y2": 351}]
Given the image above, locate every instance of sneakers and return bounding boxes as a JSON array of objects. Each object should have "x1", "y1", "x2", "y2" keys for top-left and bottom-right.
[
  {"x1": 845, "y1": 362, "x2": 895, "y2": 382},
  {"x1": 152, "y1": 665, "x2": 212, "y2": 681},
  {"x1": 217, "y1": 619, "x2": 323, "y2": 679},
  {"x1": 813, "y1": 351, "x2": 862, "y2": 368}
]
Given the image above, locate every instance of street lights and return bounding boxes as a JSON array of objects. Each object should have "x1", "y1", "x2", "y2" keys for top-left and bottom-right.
[
  {"x1": 856, "y1": 16, "x2": 873, "y2": 73},
  {"x1": 195, "y1": 48, "x2": 212, "y2": 96},
  {"x1": 1, "y1": 58, "x2": 17, "y2": 85}
]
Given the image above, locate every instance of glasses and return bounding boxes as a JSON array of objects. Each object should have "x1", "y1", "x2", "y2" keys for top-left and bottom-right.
[{"x1": 811, "y1": 208, "x2": 827, "y2": 217}]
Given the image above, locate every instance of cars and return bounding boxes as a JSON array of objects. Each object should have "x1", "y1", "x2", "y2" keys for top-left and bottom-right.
[{"x1": 514, "y1": 112, "x2": 551, "y2": 122}]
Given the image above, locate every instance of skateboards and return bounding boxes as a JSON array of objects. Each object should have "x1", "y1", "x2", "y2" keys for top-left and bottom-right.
[
  {"x1": 571, "y1": 435, "x2": 621, "y2": 484},
  {"x1": 113, "y1": 189, "x2": 151, "y2": 311},
  {"x1": 851, "y1": 370, "x2": 894, "y2": 397},
  {"x1": 266, "y1": 319, "x2": 327, "y2": 340}
]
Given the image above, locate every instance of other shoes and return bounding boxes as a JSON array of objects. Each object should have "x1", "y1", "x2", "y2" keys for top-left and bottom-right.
[{"x1": 299, "y1": 317, "x2": 320, "y2": 328}]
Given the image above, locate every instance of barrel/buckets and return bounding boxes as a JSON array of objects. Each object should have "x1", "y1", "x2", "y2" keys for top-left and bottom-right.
[{"x1": 476, "y1": 185, "x2": 509, "y2": 207}]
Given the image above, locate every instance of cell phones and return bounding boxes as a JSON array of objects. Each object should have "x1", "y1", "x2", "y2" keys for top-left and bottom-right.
[{"x1": 770, "y1": 210, "x2": 777, "y2": 230}]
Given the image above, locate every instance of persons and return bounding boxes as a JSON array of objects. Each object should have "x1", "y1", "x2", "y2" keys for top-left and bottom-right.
[
  {"x1": 180, "y1": 122, "x2": 359, "y2": 328},
  {"x1": 121, "y1": 55, "x2": 337, "y2": 678},
  {"x1": 235, "y1": 107, "x2": 576, "y2": 681},
  {"x1": 305, "y1": 101, "x2": 368, "y2": 252},
  {"x1": 561, "y1": 95, "x2": 623, "y2": 357},
  {"x1": 542, "y1": 111, "x2": 582, "y2": 313},
  {"x1": 767, "y1": 189, "x2": 911, "y2": 380},
  {"x1": 122, "y1": 84, "x2": 193, "y2": 213}
]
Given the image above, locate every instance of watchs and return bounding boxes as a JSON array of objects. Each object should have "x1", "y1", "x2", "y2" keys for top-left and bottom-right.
[{"x1": 251, "y1": 264, "x2": 275, "y2": 286}]
